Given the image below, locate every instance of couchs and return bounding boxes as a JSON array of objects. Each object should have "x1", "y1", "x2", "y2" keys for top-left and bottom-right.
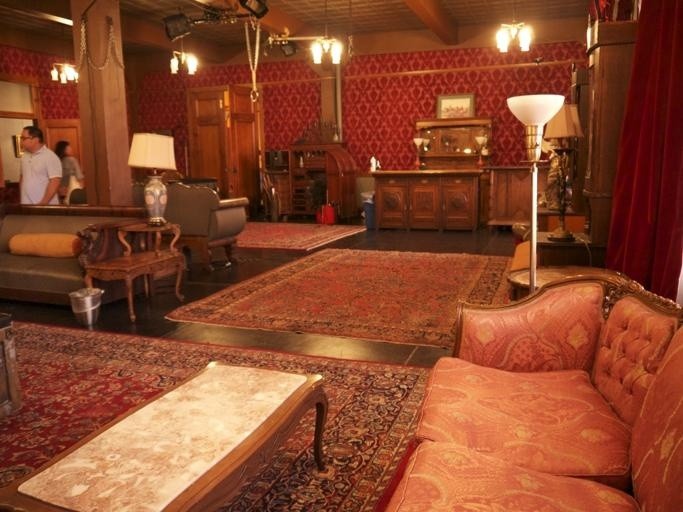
[
  {"x1": 0, "y1": 204, "x2": 147, "y2": 307},
  {"x1": 372, "y1": 273, "x2": 682, "y2": 511}
]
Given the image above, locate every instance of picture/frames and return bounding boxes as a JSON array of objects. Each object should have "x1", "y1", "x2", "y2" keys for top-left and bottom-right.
[
  {"x1": 436, "y1": 94, "x2": 475, "y2": 118},
  {"x1": 16, "y1": 135, "x2": 28, "y2": 157}
]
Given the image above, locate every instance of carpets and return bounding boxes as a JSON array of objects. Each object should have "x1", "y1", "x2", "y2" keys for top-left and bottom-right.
[
  {"x1": 235, "y1": 222, "x2": 367, "y2": 251},
  {"x1": 1, "y1": 322, "x2": 433, "y2": 511},
  {"x1": 165, "y1": 247, "x2": 514, "y2": 349}
]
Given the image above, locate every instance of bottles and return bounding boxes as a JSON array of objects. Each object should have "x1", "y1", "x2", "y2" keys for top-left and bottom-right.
[
  {"x1": 483, "y1": 134, "x2": 488, "y2": 149},
  {"x1": 370, "y1": 156, "x2": 376, "y2": 172}
]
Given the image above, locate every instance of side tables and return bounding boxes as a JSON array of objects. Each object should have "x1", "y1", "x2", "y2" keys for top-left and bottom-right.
[{"x1": 119, "y1": 222, "x2": 184, "y2": 302}]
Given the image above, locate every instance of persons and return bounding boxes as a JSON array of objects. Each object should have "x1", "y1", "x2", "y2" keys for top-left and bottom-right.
[
  {"x1": 18, "y1": 126, "x2": 63, "y2": 205},
  {"x1": 55, "y1": 141, "x2": 87, "y2": 206}
]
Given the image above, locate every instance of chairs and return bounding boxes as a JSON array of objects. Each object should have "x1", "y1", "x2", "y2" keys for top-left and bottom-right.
[{"x1": 164, "y1": 182, "x2": 249, "y2": 272}]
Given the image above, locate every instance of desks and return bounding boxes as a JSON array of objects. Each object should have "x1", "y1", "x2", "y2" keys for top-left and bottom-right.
[{"x1": 0, "y1": 313, "x2": 24, "y2": 404}]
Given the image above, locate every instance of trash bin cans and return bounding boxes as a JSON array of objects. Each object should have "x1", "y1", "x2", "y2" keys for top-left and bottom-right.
[
  {"x1": 68, "y1": 287, "x2": 102, "y2": 326},
  {"x1": 361, "y1": 190, "x2": 376, "y2": 230},
  {"x1": 1, "y1": 313, "x2": 22, "y2": 419}
]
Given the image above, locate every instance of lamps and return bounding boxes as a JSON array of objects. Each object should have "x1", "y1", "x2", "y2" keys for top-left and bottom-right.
[
  {"x1": 127, "y1": 133, "x2": 177, "y2": 225},
  {"x1": 544, "y1": 104, "x2": 585, "y2": 241},
  {"x1": 507, "y1": 94, "x2": 565, "y2": 294}
]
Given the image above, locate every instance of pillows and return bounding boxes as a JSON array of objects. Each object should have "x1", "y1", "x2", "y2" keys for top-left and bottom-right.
[{"x1": 8, "y1": 233, "x2": 79, "y2": 259}]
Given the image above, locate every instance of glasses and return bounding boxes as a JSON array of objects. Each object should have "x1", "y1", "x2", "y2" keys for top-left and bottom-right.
[{"x1": 20, "y1": 137, "x2": 34, "y2": 140}]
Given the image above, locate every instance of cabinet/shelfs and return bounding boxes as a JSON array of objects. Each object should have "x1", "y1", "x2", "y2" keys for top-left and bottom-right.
[
  {"x1": 586, "y1": 21, "x2": 637, "y2": 248},
  {"x1": 564, "y1": 64, "x2": 590, "y2": 215},
  {"x1": 262, "y1": 142, "x2": 357, "y2": 222},
  {"x1": 187, "y1": 83, "x2": 265, "y2": 221},
  {"x1": 371, "y1": 117, "x2": 548, "y2": 232}
]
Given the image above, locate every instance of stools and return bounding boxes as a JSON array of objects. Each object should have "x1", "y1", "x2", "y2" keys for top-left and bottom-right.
[{"x1": 84, "y1": 249, "x2": 185, "y2": 322}]
